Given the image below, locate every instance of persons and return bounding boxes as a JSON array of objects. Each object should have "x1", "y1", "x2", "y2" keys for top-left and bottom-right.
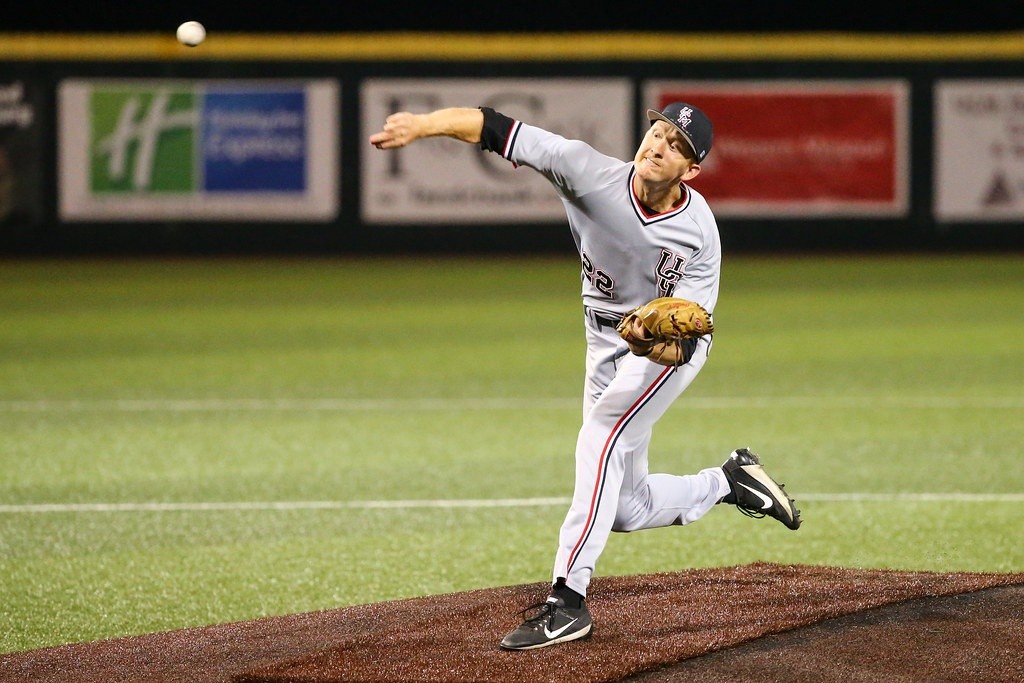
[{"x1": 369, "y1": 102, "x2": 804, "y2": 650}]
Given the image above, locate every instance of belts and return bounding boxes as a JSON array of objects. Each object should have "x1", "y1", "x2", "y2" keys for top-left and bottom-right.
[{"x1": 584, "y1": 305, "x2": 622, "y2": 330}]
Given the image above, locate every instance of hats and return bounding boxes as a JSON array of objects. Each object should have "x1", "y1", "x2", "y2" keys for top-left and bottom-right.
[{"x1": 647, "y1": 101, "x2": 714, "y2": 165}]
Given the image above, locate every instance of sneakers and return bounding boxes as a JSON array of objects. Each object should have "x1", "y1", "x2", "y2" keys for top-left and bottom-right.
[
  {"x1": 723, "y1": 448, "x2": 802, "y2": 531},
  {"x1": 500, "y1": 594, "x2": 592, "y2": 649}
]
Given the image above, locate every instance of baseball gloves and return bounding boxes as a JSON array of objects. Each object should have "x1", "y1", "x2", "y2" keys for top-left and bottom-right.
[{"x1": 615, "y1": 296, "x2": 716, "y2": 373}]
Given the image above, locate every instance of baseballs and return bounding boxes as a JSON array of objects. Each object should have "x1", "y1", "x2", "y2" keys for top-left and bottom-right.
[{"x1": 176, "y1": 20, "x2": 206, "y2": 47}]
